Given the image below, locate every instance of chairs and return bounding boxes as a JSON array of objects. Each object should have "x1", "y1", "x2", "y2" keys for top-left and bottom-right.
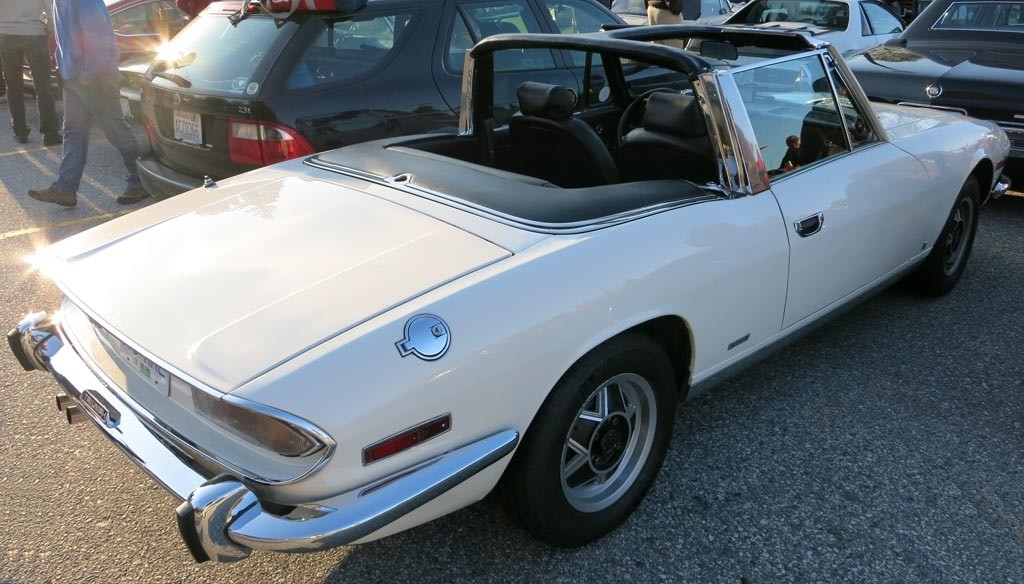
[
  {"x1": 617, "y1": 90, "x2": 717, "y2": 185},
  {"x1": 478, "y1": 22, "x2": 521, "y2": 38},
  {"x1": 509, "y1": 80, "x2": 618, "y2": 186}
]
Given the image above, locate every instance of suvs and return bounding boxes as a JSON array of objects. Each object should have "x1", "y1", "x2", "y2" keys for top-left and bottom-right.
[{"x1": 127, "y1": 0, "x2": 735, "y2": 205}]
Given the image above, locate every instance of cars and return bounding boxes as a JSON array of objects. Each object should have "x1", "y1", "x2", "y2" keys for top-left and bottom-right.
[
  {"x1": 838, "y1": 0, "x2": 1024, "y2": 200},
  {"x1": 2, "y1": 0, "x2": 1024, "y2": 211},
  {"x1": 2, "y1": 21, "x2": 1016, "y2": 569}
]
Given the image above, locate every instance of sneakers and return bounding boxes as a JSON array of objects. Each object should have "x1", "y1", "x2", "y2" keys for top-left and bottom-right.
[
  {"x1": 44, "y1": 133, "x2": 63, "y2": 145},
  {"x1": 117, "y1": 187, "x2": 149, "y2": 204},
  {"x1": 14, "y1": 127, "x2": 27, "y2": 143},
  {"x1": 28, "y1": 187, "x2": 76, "y2": 206}
]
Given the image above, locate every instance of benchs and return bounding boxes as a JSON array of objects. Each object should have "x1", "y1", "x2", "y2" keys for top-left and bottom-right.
[{"x1": 304, "y1": 46, "x2": 391, "y2": 83}]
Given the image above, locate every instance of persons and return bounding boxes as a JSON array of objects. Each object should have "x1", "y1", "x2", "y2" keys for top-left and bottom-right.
[
  {"x1": 0, "y1": 0, "x2": 63, "y2": 145},
  {"x1": 781, "y1": 135, "x2": 806, "y2": 169},
  {"x1": 28, "y1": 0, "x2": 151, "y2": 205},
  {"x1": 644, "y1": 0, "x2": 683, "y2": 48}
]
